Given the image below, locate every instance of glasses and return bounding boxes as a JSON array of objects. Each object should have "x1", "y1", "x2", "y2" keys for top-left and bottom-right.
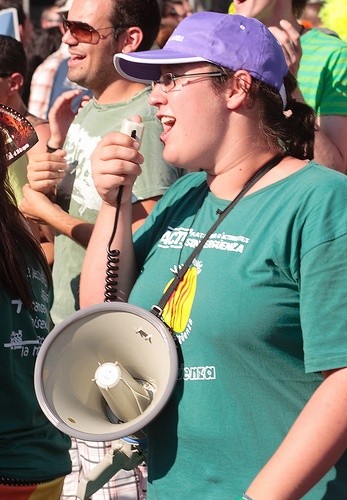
[
  {"x1": 150, "y1": 70, "x2": 231, "y2": 93},
  {"x1": 62, "y1": 20, "x2": 146, "y2": 44}
]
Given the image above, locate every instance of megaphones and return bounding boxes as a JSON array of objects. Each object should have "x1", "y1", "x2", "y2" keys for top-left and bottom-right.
[{"x1": 34, "y1": 302, "x2": 178, "y2": 500}]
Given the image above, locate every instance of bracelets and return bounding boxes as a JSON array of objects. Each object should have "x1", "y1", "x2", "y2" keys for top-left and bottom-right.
[
  {"x1": 46, "y1": 144, "x2": 58, "y2": 154},
  {"x1": 241, "y1": 493, "x2": 253, "y2": 500}
]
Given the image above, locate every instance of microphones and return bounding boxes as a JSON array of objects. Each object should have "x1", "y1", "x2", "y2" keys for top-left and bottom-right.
[{"x1": 120, "y1": 118, "x2": 144, "y2": 143}]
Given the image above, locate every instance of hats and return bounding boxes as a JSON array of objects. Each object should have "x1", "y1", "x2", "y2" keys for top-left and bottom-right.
[{"x1": 113, "y1": 12, "x2": 289, "y2": 111}]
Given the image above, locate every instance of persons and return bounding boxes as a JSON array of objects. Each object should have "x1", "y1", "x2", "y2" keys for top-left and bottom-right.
[
  {"x1": 0, "y1": 0, "x2": 347, "y2": 263},
  {"x1": 16, "y1": 0, "x2": 202, "y2": 500},
  {"x1": 80, "y1": 9, "x2": 347, "y2": 500},
  {"x1": 0, "y1": 126, "x2": 76, "y2": 500}
]
